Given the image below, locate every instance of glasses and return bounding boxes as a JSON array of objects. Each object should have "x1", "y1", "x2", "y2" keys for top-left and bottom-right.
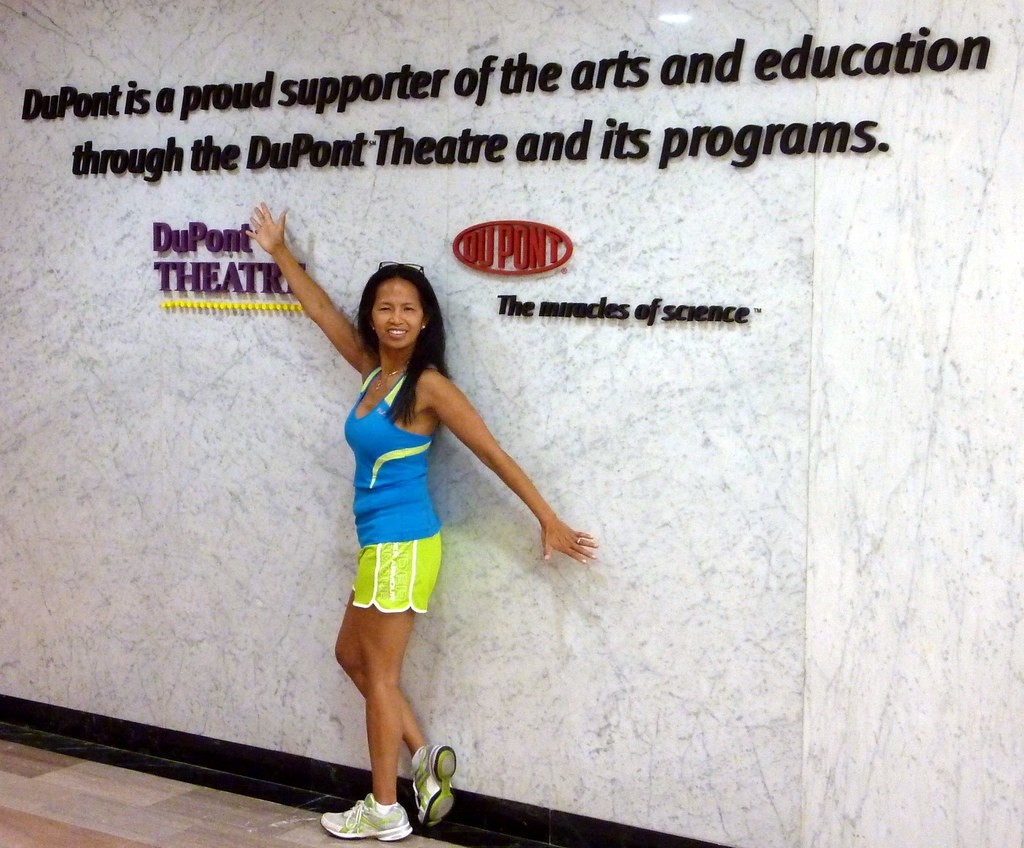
[{"x1": 379, "y1": 261, "x2": 425, "y2": 276}]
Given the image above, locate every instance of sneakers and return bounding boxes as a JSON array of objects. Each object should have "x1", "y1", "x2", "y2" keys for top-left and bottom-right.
[
  {"x1": 411, "y1": 744, "x2": 457, "y2": 827},
  {"x1": 320, "y1": 793, "x2": 413, "y2": 841}
]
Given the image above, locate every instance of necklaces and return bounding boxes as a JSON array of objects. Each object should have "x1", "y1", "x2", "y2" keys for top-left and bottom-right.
[{"x1": 376, "y1": 370, "x2": 399, "y2": 390}]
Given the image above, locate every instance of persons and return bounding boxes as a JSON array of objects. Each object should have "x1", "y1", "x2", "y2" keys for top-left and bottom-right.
[{"x1": 245, "y1": 201, "x2": 597, "y2": 841}]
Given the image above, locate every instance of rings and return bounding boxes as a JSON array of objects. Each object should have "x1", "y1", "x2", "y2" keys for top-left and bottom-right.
[{"x1": 577, "y1": 538, "x2": 580, "y2": 543}]
